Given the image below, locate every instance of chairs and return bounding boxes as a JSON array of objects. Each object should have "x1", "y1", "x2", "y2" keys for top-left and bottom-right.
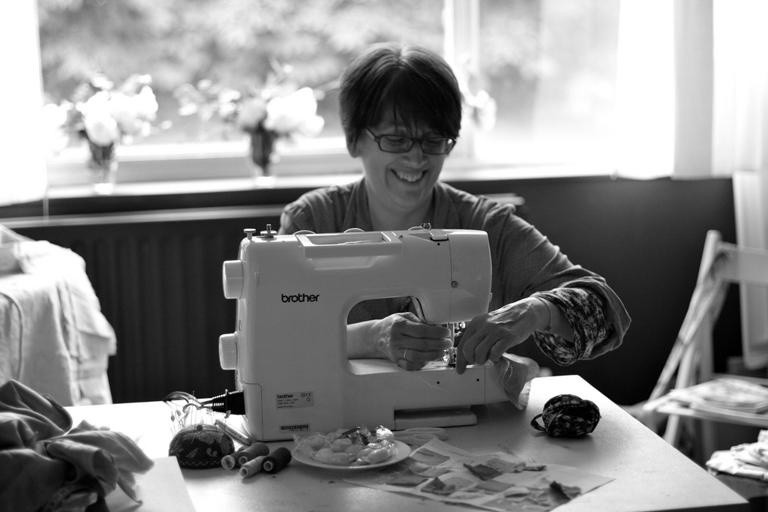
[{"x1": 619, "y1": 228, "x2": 768, "y2": 510}]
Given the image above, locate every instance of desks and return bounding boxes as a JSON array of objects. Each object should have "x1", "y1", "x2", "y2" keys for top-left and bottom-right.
[{"x1": 61, "y1": 373, "x2": 754, "y2": 510}]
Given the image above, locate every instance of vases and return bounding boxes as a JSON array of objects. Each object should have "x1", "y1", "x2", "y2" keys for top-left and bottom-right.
[
  {"x1": 242, "y1": 127, "x2": 282, "y2": 188},
  {"x1": 79, "y1": 127, "x2": 120, "y2": 193}
]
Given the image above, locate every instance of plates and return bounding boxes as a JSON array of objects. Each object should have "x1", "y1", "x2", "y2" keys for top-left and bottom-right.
[{"x1": 290, "y1": 438, "x2": 412, "y2": 471}]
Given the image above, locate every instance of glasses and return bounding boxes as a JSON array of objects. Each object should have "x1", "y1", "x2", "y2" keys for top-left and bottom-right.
[{"x1": 365, "y1": 127, "x2": 456, "y2": 156}]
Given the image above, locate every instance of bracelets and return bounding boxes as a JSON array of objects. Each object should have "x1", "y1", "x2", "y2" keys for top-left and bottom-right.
[{"x1": 535, "y1": 296, "x2": 553, "y2": 333}]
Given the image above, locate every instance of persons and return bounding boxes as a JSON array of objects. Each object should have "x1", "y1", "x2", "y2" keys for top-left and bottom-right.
[{"x1": 276, "y1": 44, "x2": 631, "y2": 376}]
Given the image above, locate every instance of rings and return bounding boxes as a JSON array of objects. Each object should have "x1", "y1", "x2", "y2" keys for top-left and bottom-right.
[{"x1": 402, "y1": 348, "x2": 409, "y2": 360}]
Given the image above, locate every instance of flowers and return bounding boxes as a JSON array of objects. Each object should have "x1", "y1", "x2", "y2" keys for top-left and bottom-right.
[{"x1": 40, "y1": 70, "x2": 327, "y2": 154}]
